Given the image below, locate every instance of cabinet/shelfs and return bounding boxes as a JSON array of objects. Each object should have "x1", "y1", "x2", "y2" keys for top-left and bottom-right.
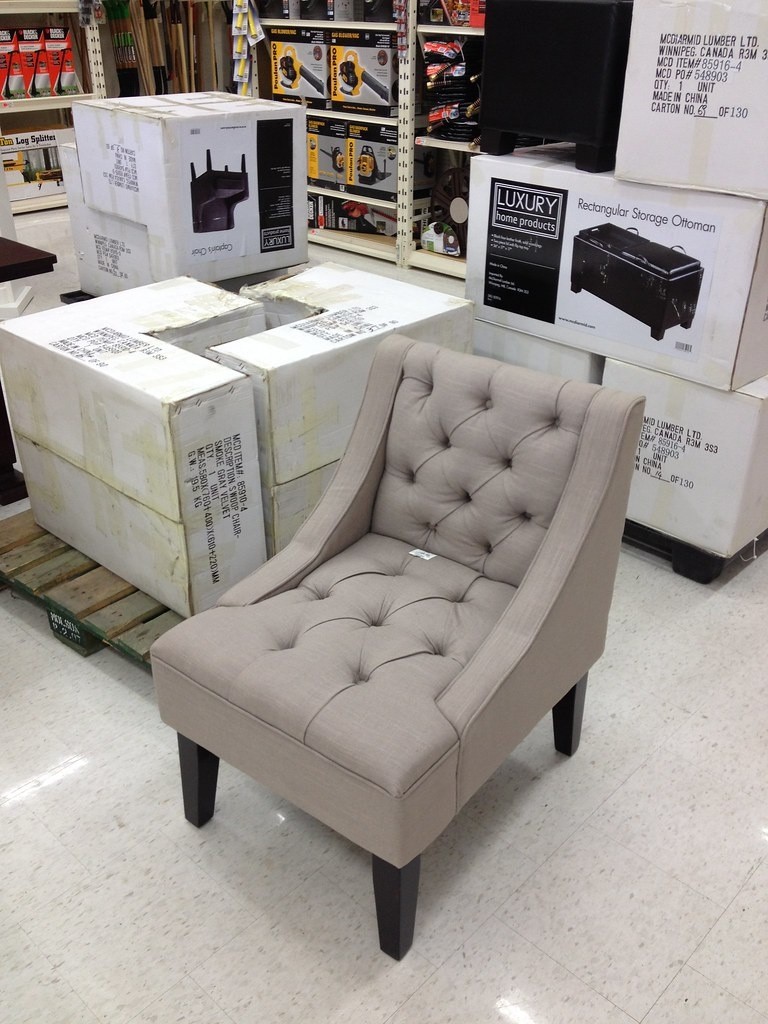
[
  {"x1": 0, "y1": 0, "x2": 107, "y2": 214},
  {"x1": 249, "y1": 0, "x2": 405, "y2": 269},
  {"x1": 401, "y1": 0, "x2": 485, "y2": 280}
]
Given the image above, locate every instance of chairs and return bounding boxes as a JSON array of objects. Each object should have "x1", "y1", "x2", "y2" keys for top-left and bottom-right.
[{"x1": 149, "y1": 334, "x2": 646, "y2": 962}]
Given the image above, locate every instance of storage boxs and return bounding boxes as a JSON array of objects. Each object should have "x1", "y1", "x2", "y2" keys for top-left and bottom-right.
[
  {"x1": 205, "y1": 261, "x2": 472, "y2": 561},
  {"x1": 58, "y1": 142, "x2": 154, "y2": 297},
  {"x1": 466, "y1": 140, "x2": 768, "y2": 392},
  {"x1": 475, "y1": 321, "x2": 606, "y2": 385},
  {"x1": 347, "y1": 121, "x2": 399, "y2": 202},
  {"x1": 306, "y1": 115, "x2": 347, "y2": 193},
  {"x1": 614, "y1": 0, "x2": 768, "y2": 202},
  {"x1": 71, "y1": 89, "x2": 309, "y2": 279},
  {"x1": 602, "y1": 358, "x2": 768, "y2": 584},
  {"x1": 330, "y1": 30, "x2": 398, "y2": 118},
  {"x1": 478, "y1": 0, "x2": 632, "y2": 173},
  {"x1": 0, "y1": 124, "x2": 75, "y2": 201},
  {"x1": 270, "y1": 27, "x2": 332, "y2": 109},
  {"x1": 0, "y1": 274, "x2": 269, "y2": 618}
]
